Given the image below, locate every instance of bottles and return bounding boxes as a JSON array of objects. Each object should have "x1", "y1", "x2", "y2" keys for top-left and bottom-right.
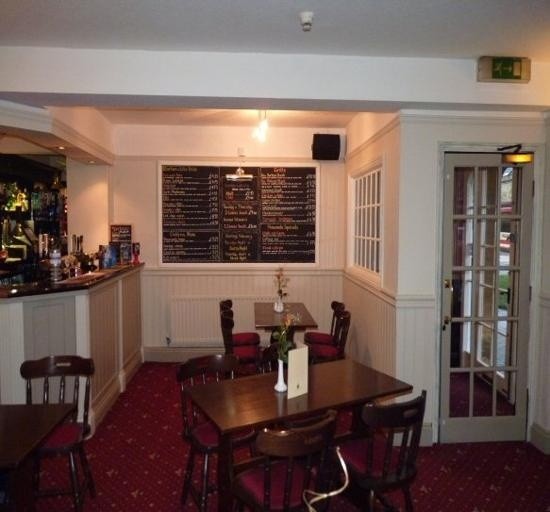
[{"x1": 49, "y1": 240, "x2": 63, "y2": 281}]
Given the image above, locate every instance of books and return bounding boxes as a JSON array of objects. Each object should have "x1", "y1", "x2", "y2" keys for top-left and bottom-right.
[{"x1": 287, "y1": 341, "x2": 309, "y2": 399}]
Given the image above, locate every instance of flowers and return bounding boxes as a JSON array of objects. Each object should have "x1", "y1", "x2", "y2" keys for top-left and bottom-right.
[
  {"x1": 274, "y1": 267, "x2": 291, "y2": 300},
  {"x1": 272, "y1": 312, "x2": 296, "y2": 364}
]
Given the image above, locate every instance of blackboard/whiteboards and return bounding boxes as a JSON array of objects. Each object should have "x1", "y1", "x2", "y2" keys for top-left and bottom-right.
[
  {"x1": 157, "y1": 159, "x2": 319, "y2": 268},
  {"x1": 110, "y1": 225, "x2": 132, "y2": 261}
]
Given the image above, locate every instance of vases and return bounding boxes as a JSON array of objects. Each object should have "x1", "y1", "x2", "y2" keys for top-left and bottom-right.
[
  {"x1": 273, "y1": 295, "x2": 284, "y2": 313},
  {"x1": 274, "y1": 358, "x2": 288, "y2": 393}
]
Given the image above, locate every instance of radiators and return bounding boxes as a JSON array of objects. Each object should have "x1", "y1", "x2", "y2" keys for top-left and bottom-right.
[{"x1": 166, "y1": 295, "x2": 281, "y2": 348}]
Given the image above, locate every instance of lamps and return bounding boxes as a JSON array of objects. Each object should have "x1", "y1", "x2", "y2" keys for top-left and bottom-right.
[
  {"x1": 496, "y1": 144, "x2": 534, "y2": 166},
  {"x1": 248, "y1": 110, "x2": 277, "y2": 146}
]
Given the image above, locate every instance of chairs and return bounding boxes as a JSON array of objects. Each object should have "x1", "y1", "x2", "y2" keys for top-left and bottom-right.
[
  {"x1": 229, "y1": 408, "x2": 339, "y2": 512},
  {"x1": 334, "y1": 390, "x2": 426, "y2": 512},
  {"x1": 20, "y1": 355, "x2": 98, "y2": 512},
  {"x1": 304, "y1": 301, "x2": 351, "y2": 364},
  {"x1": 220, "y1": 299, "x2": 264, "y2": 380},
  {"x1": 176, "y1": 355, "x2": 260, "y2": 512}
]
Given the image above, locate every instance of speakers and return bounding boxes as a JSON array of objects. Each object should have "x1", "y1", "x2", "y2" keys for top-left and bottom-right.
[{"x1": 312, "y1": 133, "x2": 340, "y2": 161}]
